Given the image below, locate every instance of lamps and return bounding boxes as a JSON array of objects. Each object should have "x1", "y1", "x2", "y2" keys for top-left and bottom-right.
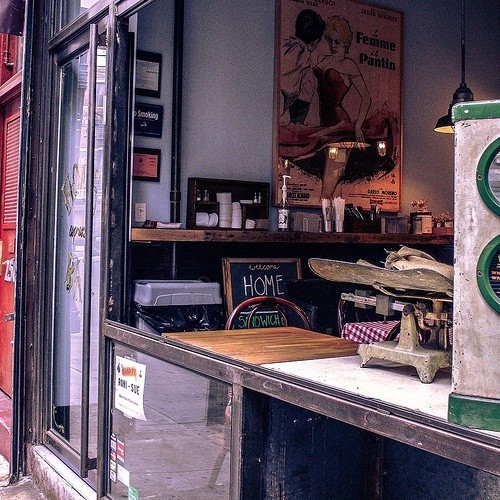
[{"x1": 434, "y1": 0, "x2": 474, "y2": 133}]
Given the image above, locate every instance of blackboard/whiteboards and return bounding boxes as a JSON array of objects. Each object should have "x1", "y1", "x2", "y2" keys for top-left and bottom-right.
[{"x1": 221, "y1": 256, "x2": 305, "y2": 330}]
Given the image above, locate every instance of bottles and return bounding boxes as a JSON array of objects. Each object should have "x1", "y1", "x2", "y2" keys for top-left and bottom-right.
[{"x1": 197, "y1": 190, "x2": 210, "y2": 201}]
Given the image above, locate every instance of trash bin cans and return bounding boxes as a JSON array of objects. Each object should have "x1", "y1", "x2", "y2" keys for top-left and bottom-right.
[{"x1": 131, "y1": 279, "x2": 228, "y2": 337}]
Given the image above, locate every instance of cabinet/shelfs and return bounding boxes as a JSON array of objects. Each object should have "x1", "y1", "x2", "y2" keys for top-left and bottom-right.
[{"x1": 185, "y1": 177, "x2": 271, "y2": 232}]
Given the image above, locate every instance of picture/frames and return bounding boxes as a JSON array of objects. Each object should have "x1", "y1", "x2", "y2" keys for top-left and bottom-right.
[{"x1": 271, "y1": 0, "x2": 403, "y2": 212}]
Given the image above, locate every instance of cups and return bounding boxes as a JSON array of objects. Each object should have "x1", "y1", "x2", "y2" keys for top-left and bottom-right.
[
  {"x1": 324, "y1": 220, "x2": 334, "y2": 232},
  {"x1": 216, "y1": 192, "x2": 242, "y2": 229},
  {"x1": 336, "y1": 220, "x2": 344, "y2": 232}
]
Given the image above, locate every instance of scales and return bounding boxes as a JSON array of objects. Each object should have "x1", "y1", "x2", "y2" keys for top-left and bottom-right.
[{"x1": 307, "y1": 257, "x2": 454, "y2": 384}]
